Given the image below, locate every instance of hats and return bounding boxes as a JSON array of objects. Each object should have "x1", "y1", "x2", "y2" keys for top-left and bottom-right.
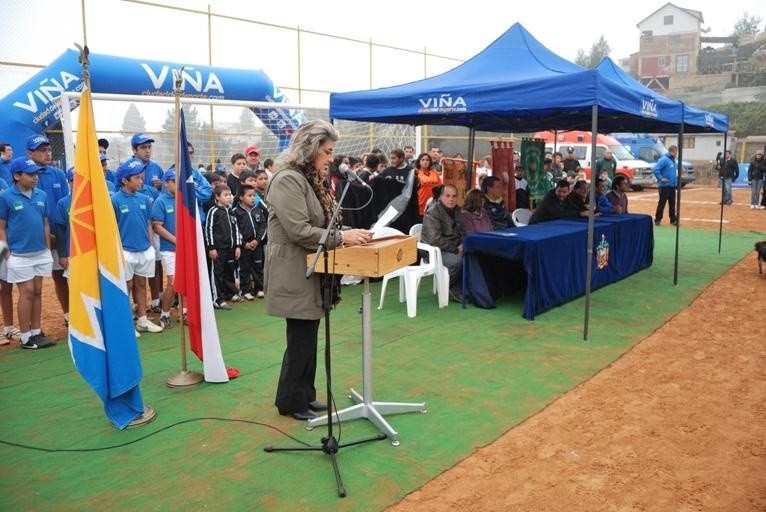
[
  {"x1": 25, "y1": 135, "x2": 51, "y2": 151},
  {"x1": 10, "y1": 156, "x2": 46, "y2": 174},
  {"x1": 98, "y1": 153, "x2": 109, "y2": 162},
  {"x1": 67, "y1": 166, "x2": 74, "y2": 180},
  {"x1": 98, "y1": 139, "x2": 109, "y2": 150},
  {"x1": 246, "y1": 144, "x2": 261, "y2": 156},
  {"x1": 132, "y1": 133, "x2": 155, "y2": 145},
  {"x1": 163, "y1": 167, "x2": 178, "y2": 180},
  {"x1": 117, "y1": 157, "x2": 147, "y2": 180}
]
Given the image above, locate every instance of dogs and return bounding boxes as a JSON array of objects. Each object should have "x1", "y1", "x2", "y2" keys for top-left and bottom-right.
[{"x1": 754, "y1": 240, "x2": 766, "y2": 275}]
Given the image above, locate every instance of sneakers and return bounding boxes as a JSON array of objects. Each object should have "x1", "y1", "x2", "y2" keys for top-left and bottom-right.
[
  {"x1": 133, "y1": 295, "x2": 189, "y2": 338},
  {"x1": 245, "y1": 293, "x2": 255, "y2": 300},
  {"x1": 448, "y1": 283, "x2": 469, "y2": 303},
  {"x1": 751, "y1": 205, "x2": 765, "y2": 209},
  {"x1": 215, "y1": 301, "x2": 230, "y2": 309},
  {"x1": 655, "y1": 220, "x2": 661, "y2": 226},
  {"x1": 672, "y1": 221, "x2": 677, "y2": 225},
  {"x1": 1, "y1": 328, "x2": 56, "y2": 350},
  {"x1": 257, "y1": 291, "x2": 264, "y2": 298},
  {"x1": 232, "y1": 294, "x2": 245, "y2": 303}
]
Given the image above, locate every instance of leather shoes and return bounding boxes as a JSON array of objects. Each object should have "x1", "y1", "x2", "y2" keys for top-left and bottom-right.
[
  {"x1": 309, "y1": 401, "x2": 328, "y2": 412},
  {"x1": 277, "y1": 408, "x2": 318, "y2": 419}
]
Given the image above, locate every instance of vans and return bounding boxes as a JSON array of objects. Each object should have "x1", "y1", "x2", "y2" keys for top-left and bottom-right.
[
  {"x1": 533, "y1": 131, "x2": 657, "y2": 193},
  {"x1": 613, "y1": 135, "x2": 696, "y2": 193}
]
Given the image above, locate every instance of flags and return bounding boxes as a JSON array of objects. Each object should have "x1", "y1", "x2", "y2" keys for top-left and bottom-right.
[
  {"x1": 175, "y1": 113, "x2": 241, "y2": 384},
  {"x1": 66, "y1": 79, "x2": 144, "y2": 428}
]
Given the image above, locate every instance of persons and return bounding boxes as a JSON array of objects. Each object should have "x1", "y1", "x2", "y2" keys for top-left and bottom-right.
[
  {"x1": 98, "y1": 133, "x2": 273, "y2": 339},
  {"x1": 1, "y1": 132, "x2": 75, "y2": 349},
  {"x1": 747, "y1": 150, "x2": 766, "y2": 209},
  {"x1": 719, "y1": 150, "x2": 739, "y2": 205},
  {"x1": 264, "y1": 119, "x2": 375, "y2": 422},
  {"x1": 716, "y1": 152, "x2": 723, "y2": 179},
  {"x1": 326, "y1": 145, "x2": 628, "y2": 302},
  {"x1": 651, "y1": 145, "x2": 678, "y2": 226}
]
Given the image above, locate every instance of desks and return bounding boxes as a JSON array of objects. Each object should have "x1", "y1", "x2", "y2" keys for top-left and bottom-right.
[{"x1": 462, "y1": 213, "x2": 653, "y2": 321}]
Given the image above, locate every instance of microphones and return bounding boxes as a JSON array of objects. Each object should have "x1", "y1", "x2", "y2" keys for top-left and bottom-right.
[{"x1": 340, "y1": 163, "x2": 368, "y2": 189}]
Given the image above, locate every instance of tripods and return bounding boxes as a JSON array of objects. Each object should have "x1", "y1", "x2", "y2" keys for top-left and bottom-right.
[{"x1": 263, "y1": 257, "x2": 387, "y2": 498}]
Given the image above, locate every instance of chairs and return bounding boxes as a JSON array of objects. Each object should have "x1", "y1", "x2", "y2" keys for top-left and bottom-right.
[
  {"x1": 372, "y1": 227, "x2": 444, "y2": 319},
  {"x1": 409, "y1": 224, "x2": 450, "y2": 309},
  {"x1": 512, "y1": 208, "x2": 533, "y2": 227}
]
